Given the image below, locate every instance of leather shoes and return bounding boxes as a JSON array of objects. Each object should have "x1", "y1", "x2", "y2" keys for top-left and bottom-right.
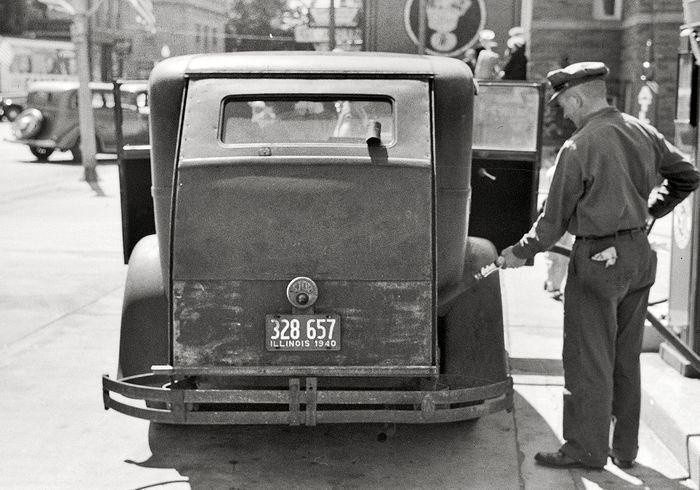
[
  {"x1": 608, "y1": 446, "x2": 633, "y2": 467},
  {"x1": 535, "y1": 451, "x2": 579, "y2": 468}
]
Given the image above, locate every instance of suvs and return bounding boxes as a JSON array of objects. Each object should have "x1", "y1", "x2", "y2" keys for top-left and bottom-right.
[{"x1": 4, "y1": 80, "x2": 151, "y2": 163}]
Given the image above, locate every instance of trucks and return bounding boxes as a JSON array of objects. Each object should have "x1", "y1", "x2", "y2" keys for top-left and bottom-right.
[{"x1": 0, "y1": 32, "x2": 77, "y2": 124}]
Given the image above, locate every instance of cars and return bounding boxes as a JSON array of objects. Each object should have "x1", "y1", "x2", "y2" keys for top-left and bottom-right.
[{"x1": 101, "y1": 51, "x2": 549, "y2": 430}]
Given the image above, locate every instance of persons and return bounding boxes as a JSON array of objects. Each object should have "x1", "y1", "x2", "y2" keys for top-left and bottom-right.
[
  {"x1": 499, "y1": 62, "x2": 700, "y2": 468},
  {"x1": 222, "y1": 100, "x2": 393, "y2": 148}
]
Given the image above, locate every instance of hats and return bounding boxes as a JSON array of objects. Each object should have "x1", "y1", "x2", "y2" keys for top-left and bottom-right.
[{"x1": 547, "y1": 62, "x2": 609, "y2": 106}]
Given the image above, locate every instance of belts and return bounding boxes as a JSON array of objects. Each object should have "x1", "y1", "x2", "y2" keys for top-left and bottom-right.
[{"x1": 576, "y1": 226, "x2": 639, "y2": 241}]
